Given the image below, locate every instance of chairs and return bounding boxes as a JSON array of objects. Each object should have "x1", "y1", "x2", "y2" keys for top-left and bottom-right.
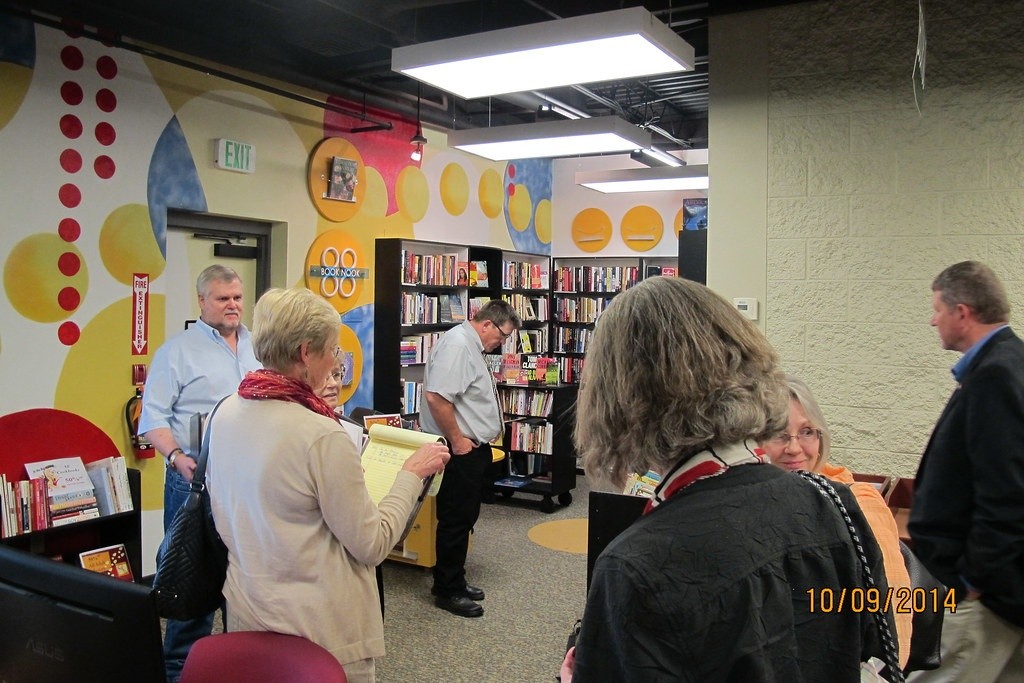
[{"x1": 177, "y1": 632, "x2": 347, "y2": 683}]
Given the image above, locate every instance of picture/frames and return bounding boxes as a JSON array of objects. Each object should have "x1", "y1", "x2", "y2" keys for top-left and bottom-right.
[{"x1": 79, "y1": 543, "x2": 135, "y2": 583}]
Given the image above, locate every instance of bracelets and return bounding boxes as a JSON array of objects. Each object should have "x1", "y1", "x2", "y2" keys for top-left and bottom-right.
[{"x1": 168, "y1": 448, "x2": 182, "y2": 467}]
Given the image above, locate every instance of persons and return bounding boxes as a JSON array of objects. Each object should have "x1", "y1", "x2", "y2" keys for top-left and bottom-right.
[
  {"x1": 139, "y1": 265, "x2": 271, "y2": 683},
  {"x1": 559, "y1": 276, "x2": 906, "y2": 683},
  {"x1": 458, "y1": 268, "x2": 468, "y2": 286},
  {"x1": 208, "y1": 289, "x2": 451, "y2": 683},
  {"x1": 759, "y1": 375, "x2": 911, "y2": 672},
  {"x1": 418, "y1": 300, "x2": 522, "y2": 618},
  {"x1": 905, "y1": 261, "x2": 1024, "y2": 683}
]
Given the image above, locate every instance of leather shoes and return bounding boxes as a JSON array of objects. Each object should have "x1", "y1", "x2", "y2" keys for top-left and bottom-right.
[
  {"x1": 435, "y1": 593, "x2": 484, "y2": 617},
  {"x1": 431, "y1": 585, "x2": 485, "y2": 601}
]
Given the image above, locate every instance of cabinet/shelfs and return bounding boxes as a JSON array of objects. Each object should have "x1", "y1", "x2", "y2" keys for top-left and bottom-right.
[{"x1": 373, "y1": 228, "x2": 707, "y2": 513}]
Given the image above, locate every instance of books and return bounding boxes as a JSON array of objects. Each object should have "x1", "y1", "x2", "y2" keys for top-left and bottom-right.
[
  {"x1": 400, "y1": 249, "x2": 678, "y2": 455},
  {"x1": 79, "y1": 544, "x2": 135, "y2": 583},
  {"x1": 0, "y1": 455, "x2": 134, "y2": 539}
]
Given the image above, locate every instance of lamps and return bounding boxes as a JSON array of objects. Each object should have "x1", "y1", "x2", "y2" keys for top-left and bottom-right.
[{"x1": 392, "y1": 1, "x2": 709, "y2": 195}]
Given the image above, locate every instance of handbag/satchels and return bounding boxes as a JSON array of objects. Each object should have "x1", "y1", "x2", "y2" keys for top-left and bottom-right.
[{"x1": 150, "y1": 393, "x2": 230, "y2": 623}]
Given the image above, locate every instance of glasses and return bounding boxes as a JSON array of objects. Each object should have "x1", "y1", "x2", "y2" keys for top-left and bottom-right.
[
  {"x1": 772, "y1": 424, "x2": 821, "y2": 446},
  {"x1": 489, "y1": 319, "x2": 511, "y2": 340},
  {"x1": 325, "y1": 343, "x2": 343, "y2": 358},
  {"x1": 325, "y1": 367, "x2": 344, "y2": 381}
]
